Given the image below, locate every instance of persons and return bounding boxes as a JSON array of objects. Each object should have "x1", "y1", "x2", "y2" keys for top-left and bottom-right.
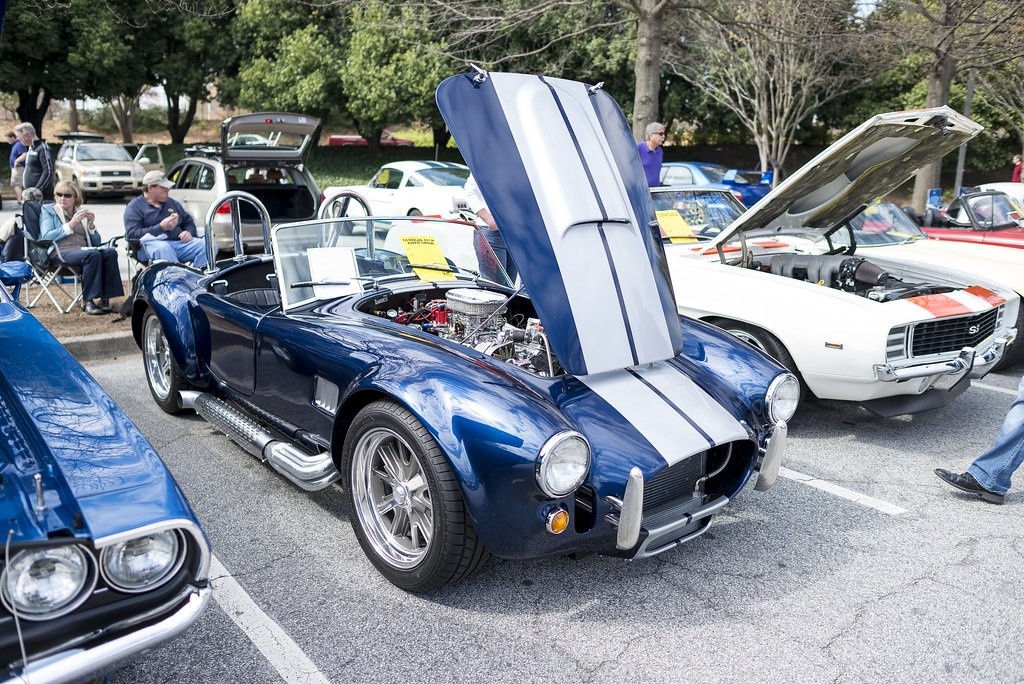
[
  {"x1": 638, "y1": 123, "x2": 665, "y2": 187},
  {"x1": 6, "y1": 132, "x2": 27, "y2": 209},
  {"x1": 15, "y1": 121, "x2": 53, "y2": 190},
  {"x1": 933, "y1": 375, "x2": 1024, "y2": 503},
  {"x1": 1012, "y1": 154, "x2": 1024, "y2": 183},
  {"x1": 123, "y1": 170, "x2": 218, "y2": 267},
  {"x1": 0, "y1": 187, "x2": 43, "y2": 243},
  {"x1": 464, "y1": 174, "x2": 518, "y2": 287},
  {"x1": 37, "y1": 179, "x2": 124, "y2": 315}
]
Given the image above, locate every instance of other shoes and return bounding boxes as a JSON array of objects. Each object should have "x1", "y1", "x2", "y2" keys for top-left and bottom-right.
[
  {"x1": 98, "y1": 302, "x2": 114, "y2": 313},
  {"x1": 10, "y1": 202, "x2": 22, "y2": 209},
  {"x1": 82, "y1": 303, "x2": 103, "y2": 314}
]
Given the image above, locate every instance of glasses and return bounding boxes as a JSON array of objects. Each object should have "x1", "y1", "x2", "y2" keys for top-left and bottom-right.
[
  {"x1": 652, "y1": 131, "x2": 664, "y2": 135},
  {"x1": 55, "y1": 192, "x2": 76, "y2": 198}
]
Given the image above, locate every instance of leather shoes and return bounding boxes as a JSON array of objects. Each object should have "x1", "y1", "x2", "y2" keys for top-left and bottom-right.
[{"x1": 934, "y1": 468, "x2": 1005, "y2": 505}]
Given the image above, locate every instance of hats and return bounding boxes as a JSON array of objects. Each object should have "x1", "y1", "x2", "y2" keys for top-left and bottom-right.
[{"x1": 142, "y1": 170, "x2": 176, "y2": 189}]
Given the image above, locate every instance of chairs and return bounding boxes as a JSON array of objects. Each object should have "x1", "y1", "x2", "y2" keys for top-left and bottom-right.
[
  {"x1": 229, "y1": 286, "x2": 281, "y2": 305},
  {"x1": 1, "y1": 214, "x2": 142, "y2": 315}
]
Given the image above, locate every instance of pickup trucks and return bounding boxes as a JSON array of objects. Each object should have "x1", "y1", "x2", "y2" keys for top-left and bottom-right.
[{"x1": 328, "y1": 127, "x2": 416, "y2": 148}]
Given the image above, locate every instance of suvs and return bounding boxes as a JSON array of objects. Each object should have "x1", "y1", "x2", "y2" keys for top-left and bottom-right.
[{"x1": 165, "y1": 110, "x2": 333, "y2": 257}]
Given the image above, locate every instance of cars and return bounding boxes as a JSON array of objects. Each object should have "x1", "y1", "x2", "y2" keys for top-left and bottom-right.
[
  {"x1": 320, "y1": 160, "x2": 491, "y2": 228},
  {"x1": 648, "y1": 105, "x2": 1021, "y2": 419},
  {"x1": 0, "y1": 256, "x2": 213, "y2": 684},
  {"x1": 52, "y1": 132, "x2": 166, "y2": 206},
  {"x1": 227, "y1": 133, "x2": 277, "y2": 147},
  {"x1": 657, "y1": 160, "x2": 1024, "y2": 279},
  {"x1": 117, "y1": 62, "x2": 802, "y2": 595}
]
